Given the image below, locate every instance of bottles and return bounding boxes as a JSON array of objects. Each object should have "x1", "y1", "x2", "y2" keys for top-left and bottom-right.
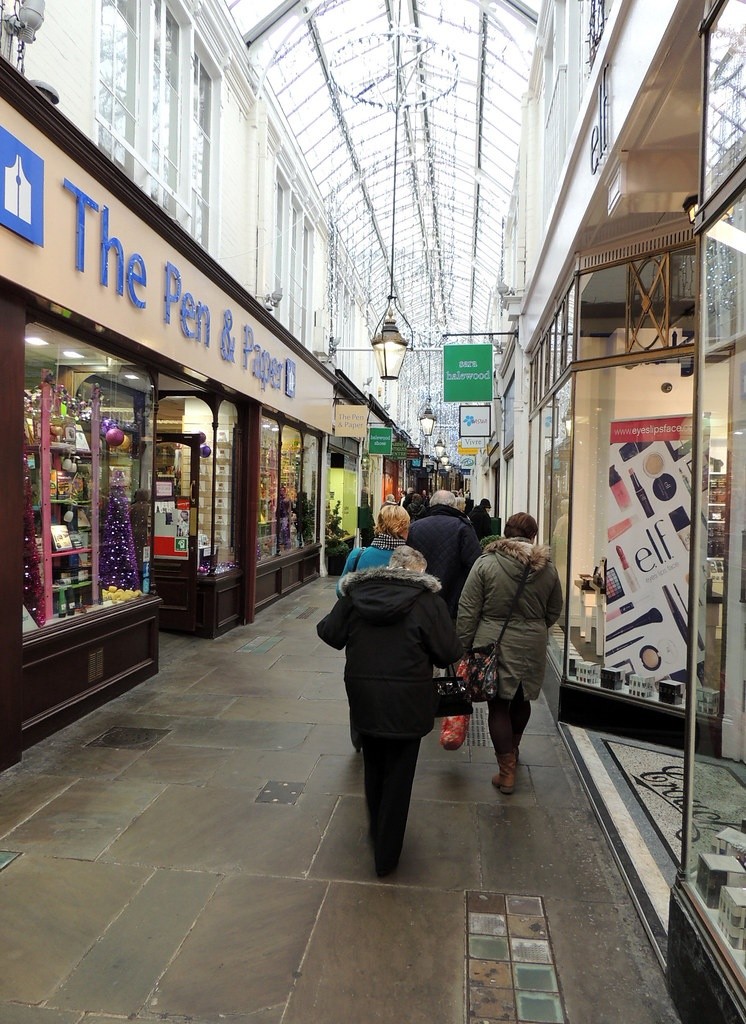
[
  {"x1": 58, "y1": 588, "x2": 66, "y2": 618},
  {"x1": 67, "y1": 587, "x2": 75, "y2": 616}
]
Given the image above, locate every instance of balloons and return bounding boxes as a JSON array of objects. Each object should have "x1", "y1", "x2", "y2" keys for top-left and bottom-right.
[
  {"x1": 199, "y1": 432, "x2": 206, "y2": 445},
  {"x1": 101, "y1": 419, "x2": 130, "y2": 449},
  {"x1": 200, "y1": 446, "x2": 211, "y2": 458}
]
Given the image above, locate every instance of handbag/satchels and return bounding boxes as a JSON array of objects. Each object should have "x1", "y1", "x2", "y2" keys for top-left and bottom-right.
[
  {"x1": 441, "y1": 659, "x2": 468, "y2": 751},
  {"x1": 456, "y1": 645, "x2": 498, "y2": 702},
  {"x1": 432, "y1": 664, "x2": 474, "y2": 718}
]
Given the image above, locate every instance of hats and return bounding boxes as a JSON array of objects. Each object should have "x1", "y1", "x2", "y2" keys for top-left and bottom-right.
[{"x1": 480, "y1": 499, "x2": 491, "y2": 508}]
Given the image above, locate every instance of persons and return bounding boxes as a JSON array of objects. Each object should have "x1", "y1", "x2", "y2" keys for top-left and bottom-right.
[
  {"x1": 411, "y1": 488, "x2": 481, "y2": 608},
  {"x1": 279, "y1": 487, "x2": 292, "y2": 549},
  {"x1": 457, "y1": 512, "x2": 563, "y2": 795},
  {"x1": 380, "y1": 486, "x2": 491, "y2": 542},
  {"x1": 317, "y1": 544, "x2": 467, "y2": 875},
  {"x1": 181, "y1": 511, "x2": 189, "y2": 524},
  {"x1": 335, "y1": 504, "x2": 413, "y2": 753}
]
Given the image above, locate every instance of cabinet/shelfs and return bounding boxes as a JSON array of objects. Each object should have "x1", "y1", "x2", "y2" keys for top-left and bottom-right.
[
  {"x1": 24, "y1": 443, "x2": 92, "y2": 623},
  {"x1": 707, "y1": 472, "x2": 725, "y2": 602}
]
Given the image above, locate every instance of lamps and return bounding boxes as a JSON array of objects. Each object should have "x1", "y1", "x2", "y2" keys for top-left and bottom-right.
[
  {"x1": 434, "y1": 351, "x2": 453, "y2": 472},
  {"x1": 421, "y1": 284, "x2": 437, "y2": 436},
  {"x1": 370, "y1": 0, "x2": 410, "y2": 381}
]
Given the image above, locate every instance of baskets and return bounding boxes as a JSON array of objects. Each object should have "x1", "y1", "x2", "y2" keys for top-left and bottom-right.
[{"x1": 88, "y1": 645, "x2": 103, "y2": 683}]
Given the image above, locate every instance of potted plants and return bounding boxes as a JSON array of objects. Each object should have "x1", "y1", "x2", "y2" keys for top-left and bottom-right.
[
  {"x1": 326, "y1": 539, "x2": 350, "y2": 576},
  {"x1": 360, "y1": 507, "x2": 376, "y2": 547}
]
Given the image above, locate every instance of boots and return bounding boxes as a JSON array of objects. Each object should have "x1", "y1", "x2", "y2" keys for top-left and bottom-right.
[{"x1": 492, "y1": 752, "x2": 517, "y2": 794}]
